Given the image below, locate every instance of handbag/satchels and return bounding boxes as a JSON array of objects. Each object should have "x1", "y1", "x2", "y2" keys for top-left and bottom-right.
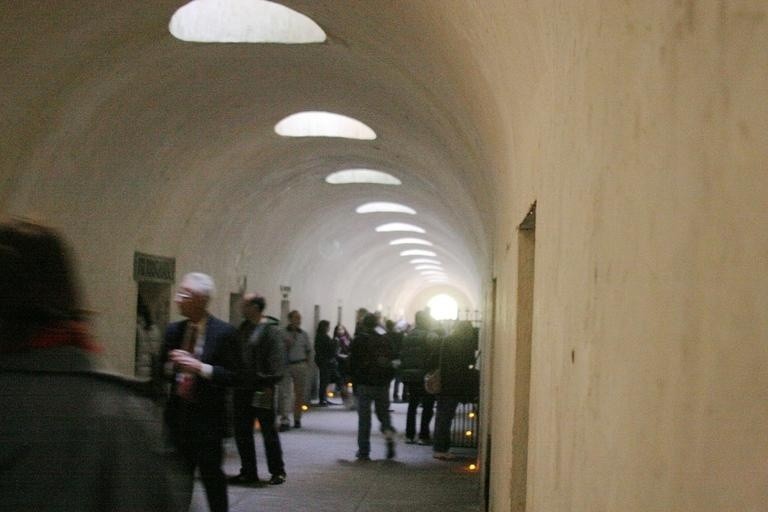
[{"x1": 423, "y1": 366, "x2": 442, "y2": 395}]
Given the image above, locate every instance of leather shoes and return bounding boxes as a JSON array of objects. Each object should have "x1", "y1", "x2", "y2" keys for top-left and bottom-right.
[
  {"x1": 225, "y1": 471, "x2": 260, "y2": 485},
  {"x1": 269, "y1": 471, "x2": 286, "y2": 485}
]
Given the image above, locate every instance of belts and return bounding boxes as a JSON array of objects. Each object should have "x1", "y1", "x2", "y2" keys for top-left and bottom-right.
[{"x1": 287, "y1": 358, "x2": 309, "y2": 364}]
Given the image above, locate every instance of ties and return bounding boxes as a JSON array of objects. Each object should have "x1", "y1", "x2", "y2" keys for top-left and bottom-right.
[{"x1": 175, "y1": 326, "x2": 203, "y2": 401}]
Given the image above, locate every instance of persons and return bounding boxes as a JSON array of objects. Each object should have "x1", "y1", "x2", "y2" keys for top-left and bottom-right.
[
  {"x1": 135, "y1": 271, "x2": 313, "y2": 510},
  {"x1": 313, "y1": 307, "x2": 477, "y2": 461},
  {"x1": 1, "y1": 219, "x2": 178, "y2": 510}
]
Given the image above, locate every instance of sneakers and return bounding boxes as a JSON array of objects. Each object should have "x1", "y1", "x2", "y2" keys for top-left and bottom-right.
[
  {"x1": 279, "y1": 420, "x2": 301, "y2": 432},
  {"x1": 403, "y1": 437, "x2": 433, "y2": 445},
  {"x1": 355, "y1": 441, "x2": 396, "y2": 460},
  {"x1": 393, "y1": 398, "x2": 406, "y2": 403},
  {"x1": 430, "y1": 450, "x2": 459, "y2": 460}
]
